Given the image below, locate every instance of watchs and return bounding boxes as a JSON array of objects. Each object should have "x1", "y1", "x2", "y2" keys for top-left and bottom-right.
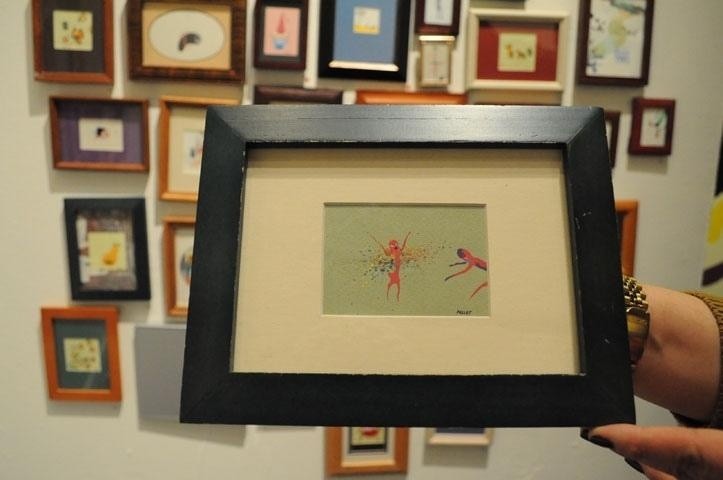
[{"x1": 617, "y1": 273, "x2": 651, "y2": 372}]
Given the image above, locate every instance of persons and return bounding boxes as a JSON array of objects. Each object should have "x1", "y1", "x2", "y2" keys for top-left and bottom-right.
[{"x1": 579, "y1": 272, "x2": 723, "y2": 480}]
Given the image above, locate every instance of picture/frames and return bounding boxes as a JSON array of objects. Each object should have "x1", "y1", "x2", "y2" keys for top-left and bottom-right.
[
  {"x1": 575, "y1": 0, "x2": 654, "y2": 88},
  {"x1": 157, "y1": 95, "x2": 243, "y2": 204},
  {"x1": 161, "y1": 214, "x2": 196, "y2": 316},
  {"x1": 628, "y1": 93, "x2": 675, "y2": 157},
  {"x1": 255, "y1": 0, "x2": 311, "y2": 71},
  {"x1": 416, "y1": 36, "x2": 457, "y2": 86},
  {"x1": 425, "y1": 426, "x2": 492, "y2": 446},
  {"x1": 40, "y1": 306, "x2": 121, "y2": 402},
  {"x1": 251, "y1": 84, "x2": 344, "y2": 104},
  {"x1": 49, "y1": 95, "x2": 149, "y2": 173},
  {"x1": 62, "y1": 198, "x2": 150, "y2": 302},
  {"x1": 352, "y1": 90, "x2": 470, "y2": 105},
  {"x1": 31, "y1": 0, "x2": 115, "y2": 84},
  {"x1": 317, "y1": 0, "x2": 410, "y2": 83},
  {"x1": 126, "y1": 0, "x2": 247, "y2": 80},
  {"x1": 179, "y1": 105, "x2": 638, "y2": 425},
  {"x1": 323, "y1": 425, "x2": 408, "y2": 475},
  {"x1": 613, "y1": 198, "x2": 641, "y2": 281},
  {"x1": 467, "y1": 8, "x2": 572, "y2": 92},
  {"x1": 415, "y1": 1, "x2": 460, "y2": 35}
]
